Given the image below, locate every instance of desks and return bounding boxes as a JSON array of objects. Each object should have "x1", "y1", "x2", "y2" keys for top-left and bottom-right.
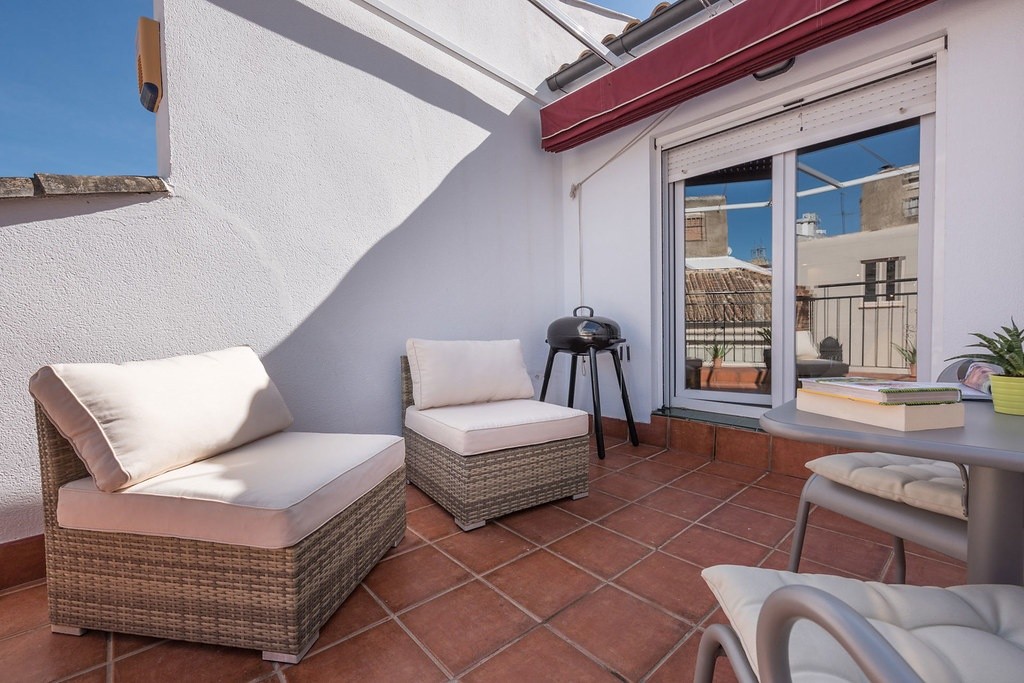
[{"x1": 760, "y1": 398, "x2": 1024, "y2": 590}]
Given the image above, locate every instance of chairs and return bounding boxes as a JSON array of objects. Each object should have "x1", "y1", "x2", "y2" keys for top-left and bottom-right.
[
  {"x1": 693, "y1": 565, "x2": 1024, "y2": 683},
  {"x1": 788, "y1": 358, "x2": 1003, "y2": 585}
]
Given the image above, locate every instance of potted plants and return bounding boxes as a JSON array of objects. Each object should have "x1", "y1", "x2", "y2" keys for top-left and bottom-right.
[
  {"x1": 944, "y1": 316, "x2": 1024, "y2": 415},
  {"x1": 894, "y1": 338, "x2": 917, "y2": 376},
  {"x1": 757, "y1": 328, "x2": 771, "y2": 369},
  {"x1": 707, "y1": 342, "x2": 732, "y2": 367}
]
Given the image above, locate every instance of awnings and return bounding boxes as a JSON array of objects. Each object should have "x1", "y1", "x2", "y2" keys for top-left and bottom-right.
[{"x1": 538, "y1": 1, "x2": 936, "y2": 155}]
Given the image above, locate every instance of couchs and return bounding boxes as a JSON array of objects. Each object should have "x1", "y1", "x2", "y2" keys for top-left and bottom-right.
[
  {"x1": 400, "y1": 338, "x2": 591, "y2": 530},
  {"x1": 796, "y1": 331, "x2": 849, "y2": 378},
  {"x1": 28, "y1": 346, "x2": 408, "y2": 664}
]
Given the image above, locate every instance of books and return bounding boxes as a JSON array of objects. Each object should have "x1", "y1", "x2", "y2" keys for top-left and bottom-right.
[
  {"x1": 796, "y1": 388, "x2": 965, "y2": 432},
  {"x1": 930, "y1": 358, "x2": 1005, "y2": 400},
  {"x1": 797, "y1": 376, "x2": 963, "y2": 406}
]
